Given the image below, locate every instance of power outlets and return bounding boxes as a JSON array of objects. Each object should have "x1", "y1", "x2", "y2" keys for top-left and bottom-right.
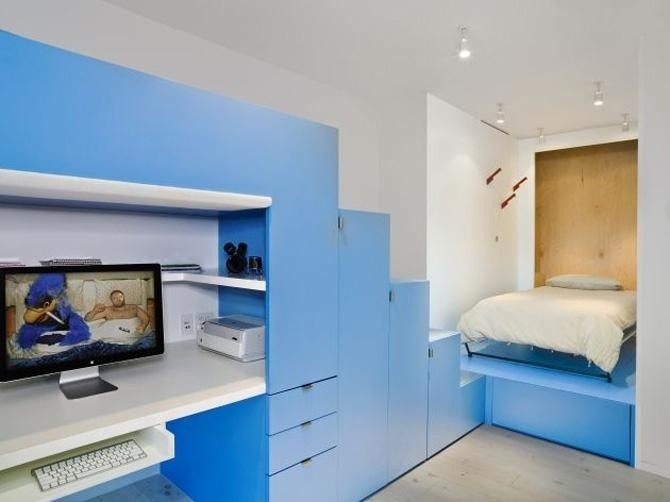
[{"x1": 180, "y1": 313, "x2": 194, "y2": 337}]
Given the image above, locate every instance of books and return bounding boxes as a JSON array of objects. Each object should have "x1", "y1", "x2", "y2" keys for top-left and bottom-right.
[
  {"x1": 39, "y1": 257, "x2": 103, "y2": 268},
  {"x1": 158, "y1": 263, "x2": 205, "y2": 274},
  {"x1": 0, "y1": 257, "x2": 29, "y2": 268}
]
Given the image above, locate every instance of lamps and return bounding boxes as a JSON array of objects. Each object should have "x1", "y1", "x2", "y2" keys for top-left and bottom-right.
[
  {"x1": 594, "y1": 80, "x2": 605, "y2": 106},
  {"x1": 496, "y1": 104, "x2": 505, "y2": 123},
  {"x1": 538, "y1": 128, "x2": 546, "y2": 146},
  {"x1": 458, "y1": 23, "x2": 470, "y2": 58},
  {"x1": 621, "y1": 113, "x2": 630, "y2": 132}
]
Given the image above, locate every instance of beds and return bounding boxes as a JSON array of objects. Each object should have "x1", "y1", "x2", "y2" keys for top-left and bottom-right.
[{"x1": 457, "y1": 285, "x2": 637, "y2": 383}]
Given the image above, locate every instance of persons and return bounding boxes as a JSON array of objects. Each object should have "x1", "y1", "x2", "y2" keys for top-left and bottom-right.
[{"x1": 85, "y1": 289, "x2": 150, "y2": 337}]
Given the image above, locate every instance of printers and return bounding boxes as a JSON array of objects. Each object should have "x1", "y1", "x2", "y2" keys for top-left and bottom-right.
[{"x1": 197, "y1": 313, "x2": 266, "y2": 362}]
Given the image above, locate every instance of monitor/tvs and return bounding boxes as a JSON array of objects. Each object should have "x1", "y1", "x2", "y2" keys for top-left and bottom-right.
[{"x1": 0, "y1": 263, "x2": 164, "y2": 399}]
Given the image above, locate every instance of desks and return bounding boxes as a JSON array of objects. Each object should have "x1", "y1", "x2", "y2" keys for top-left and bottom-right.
[{"x1": 0, "y1": 339, "x2": 268, "y2": 502}]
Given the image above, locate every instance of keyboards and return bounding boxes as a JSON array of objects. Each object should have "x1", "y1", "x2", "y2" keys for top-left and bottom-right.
[{"x1": 30, "y1": 439, "x2": 147, "y2": 491}]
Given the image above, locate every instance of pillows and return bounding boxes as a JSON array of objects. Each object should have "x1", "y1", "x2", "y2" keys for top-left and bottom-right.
[{"x1": 544, "y1": 274, "x2": 624, "y2": 290}]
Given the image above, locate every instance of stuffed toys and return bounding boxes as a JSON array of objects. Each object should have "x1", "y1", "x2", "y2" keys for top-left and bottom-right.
[{"x1": 14, "y1": 273, "x2": 89, "y2": 354}]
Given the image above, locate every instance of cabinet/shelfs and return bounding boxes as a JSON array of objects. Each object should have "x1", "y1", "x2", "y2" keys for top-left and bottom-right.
[{"x1": 160, "y1": 107, "x2": 338, "y2": 502}]
